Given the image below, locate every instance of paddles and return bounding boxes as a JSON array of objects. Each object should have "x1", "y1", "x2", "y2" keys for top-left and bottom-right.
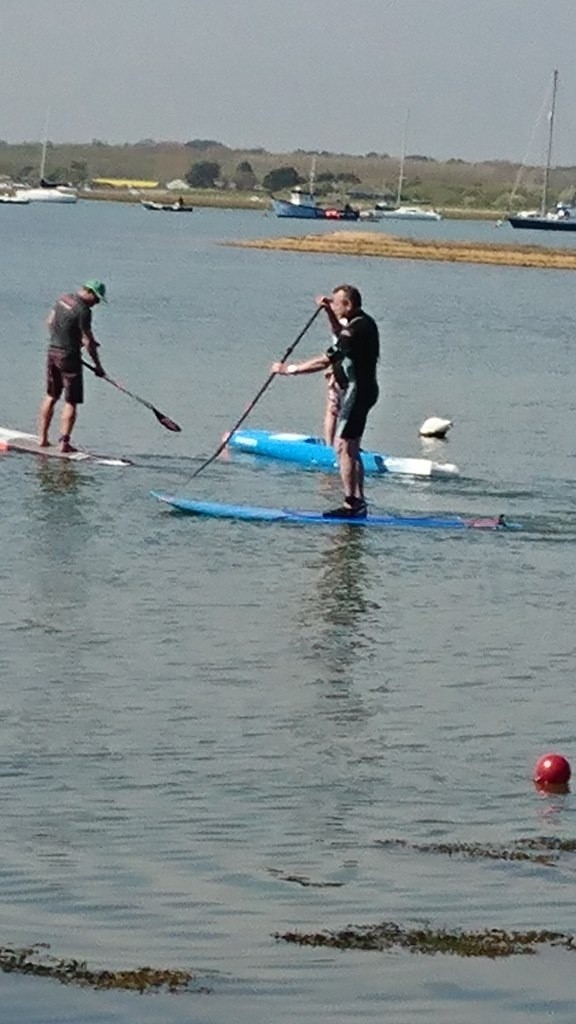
[
  {"x1": 189, "y1": 300, "x2": 331, "y2": 477},
  {"x1": 81, "y1": 359, "x2": 184, "y2": 432}
]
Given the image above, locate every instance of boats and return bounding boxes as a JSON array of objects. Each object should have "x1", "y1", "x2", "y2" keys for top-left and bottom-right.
[
  {"x1": 13, "y1": 107, "x2": 81, "y2": 205},
  {"x1": 223, "y1": 429, "x2": 462, "y2": 479},
  {"x1": 138, "y1": 199, "x2": 193, "y2": 212},
  {"x1": 0, "y1": 193, "x2": 30, "y2": 205},
  {"x1": 266, "y1": 153, "x2": 360, "y2": 222}
]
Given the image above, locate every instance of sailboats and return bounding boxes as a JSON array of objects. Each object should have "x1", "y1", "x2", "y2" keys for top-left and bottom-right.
[
  {"x1": 501, "y1": 67, "x2": 576, "y2": 231},
  {"x1": 358, "y1": 108, "x2": 444, "y2": 223}
]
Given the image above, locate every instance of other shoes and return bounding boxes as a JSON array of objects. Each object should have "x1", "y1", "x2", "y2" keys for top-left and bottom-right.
[{"x1": 321, "y1": 502, "x2": 368, "y2": 518}]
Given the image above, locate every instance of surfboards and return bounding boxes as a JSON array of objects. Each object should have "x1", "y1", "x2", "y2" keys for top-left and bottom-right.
[
  {"x1": 0, "y1": 427, "x2": 134, "y2": 467},
  {"x1": 149, "y1": 491, "x2": 522, "y2": 531}
]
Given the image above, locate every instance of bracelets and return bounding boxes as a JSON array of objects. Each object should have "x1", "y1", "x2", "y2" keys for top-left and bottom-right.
[{"x1": 287, "y1": 365, "x2": 297, "y2": 375}]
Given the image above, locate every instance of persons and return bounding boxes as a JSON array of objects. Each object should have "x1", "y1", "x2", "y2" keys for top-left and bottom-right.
[
  {"x1": 38, "y1": 279, "x2": 107, "y2": 454},
  {"x1": 270, "y1": 284, "x2": 379, "y2": 520}
]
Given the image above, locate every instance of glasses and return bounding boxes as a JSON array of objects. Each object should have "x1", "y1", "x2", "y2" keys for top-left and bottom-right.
[{"x1": 92, "y1": 291, "x2": 99, "y2": 303}]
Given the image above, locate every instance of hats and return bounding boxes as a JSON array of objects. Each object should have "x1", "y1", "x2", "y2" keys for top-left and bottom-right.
[{"x1": 82, "y1": 279, "x2": 109, "y2": 304}]
